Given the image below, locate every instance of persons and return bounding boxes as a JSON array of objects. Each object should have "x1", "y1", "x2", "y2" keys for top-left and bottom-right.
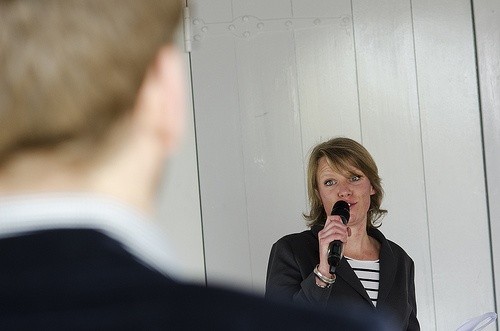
[
  {"x1": 263, "y1": 136, "x2": 422, "y2": 331},
  {"x1": 0, "y1": 0, "x2": 403, "y2": 331}
]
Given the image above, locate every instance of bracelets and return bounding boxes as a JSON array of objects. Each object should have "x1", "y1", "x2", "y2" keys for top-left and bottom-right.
[{"x1": 313, "y1": 263, "x2": 337, "y2": 284}]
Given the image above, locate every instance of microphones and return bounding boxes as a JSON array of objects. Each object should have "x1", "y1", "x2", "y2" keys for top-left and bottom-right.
[{"x1": 327, "y1": 200, "x2": 351, "y2": 274}]
